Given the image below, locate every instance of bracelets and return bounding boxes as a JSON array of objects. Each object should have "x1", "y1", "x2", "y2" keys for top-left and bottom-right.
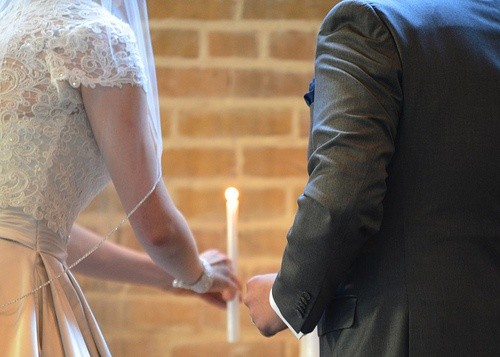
[{"x1": 169, "y1": 256, "x2": 215, "y2": 294}]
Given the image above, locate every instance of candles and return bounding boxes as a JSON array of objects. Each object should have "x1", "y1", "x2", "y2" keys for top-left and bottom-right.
[{"x1": 222, "y1": 186, "x2": 240, "y2": 344}]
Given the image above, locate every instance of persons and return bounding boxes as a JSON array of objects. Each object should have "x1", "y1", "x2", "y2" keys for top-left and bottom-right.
[
  {"x1": 0, "y1": 0, "x2": 240, "y2": 356},
  {"x1": 237, "y1": 1, "x2": 500, "y2": 357}
]
